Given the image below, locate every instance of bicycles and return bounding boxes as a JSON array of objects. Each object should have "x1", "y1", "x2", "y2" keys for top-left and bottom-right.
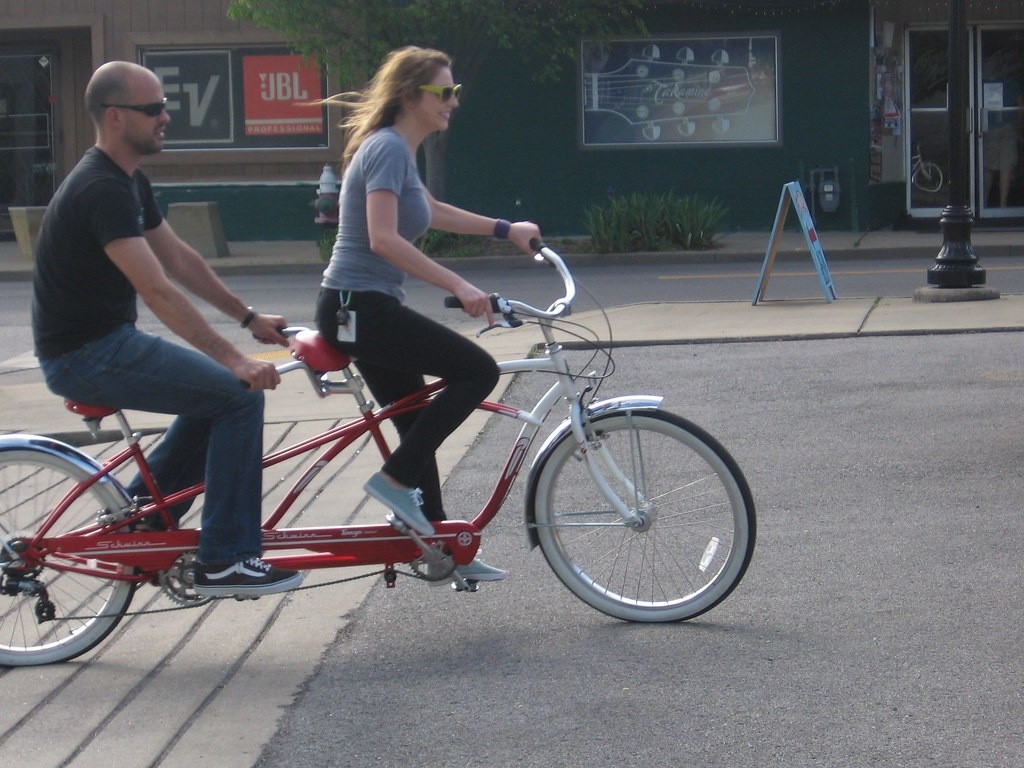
[
  {"x1": 0, "y1": 238, "x2": 759, "y2": 666},
  {"x1": 911, "y1": 144, "x2": 944, "y2": 195}
]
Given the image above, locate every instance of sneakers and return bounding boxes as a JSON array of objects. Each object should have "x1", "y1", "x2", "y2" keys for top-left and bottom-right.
[
  {"x1": 362, "y1": 471, "x2": 435, "y2": 536},
  {"x1": 192, "y1": 559, "x2": 301, "y2": 595},
  {"x1": 426, "y1": 555, "x2": 508, "y2": 586}
]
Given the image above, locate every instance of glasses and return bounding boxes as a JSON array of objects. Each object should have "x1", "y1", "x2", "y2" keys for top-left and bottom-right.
[
  {"x1": 100, "y1": 98, "x2": 168, "y2": 118},
  {"x1": 419, "y1": 84, "x2": 463, "y2": 103}
]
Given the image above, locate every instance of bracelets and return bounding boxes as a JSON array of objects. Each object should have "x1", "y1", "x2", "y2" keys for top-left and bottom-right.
[
  {"x1": 240, "y1": 306, "x2": 257, "y2": 328},
  {"x1": 495, "y1": 219, "x2": 511, "y2": 239}
]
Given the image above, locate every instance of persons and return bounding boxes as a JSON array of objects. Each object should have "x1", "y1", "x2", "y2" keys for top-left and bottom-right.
[
  {"x1": 984, "y1": 59, "x2": 1024, "y2": 208},
  {"x1": 31, "y1": 61, "x2": 304, "y2": 595},
  {"x1": 314, "y1": 47, "x2": 543, "y2": 581}
]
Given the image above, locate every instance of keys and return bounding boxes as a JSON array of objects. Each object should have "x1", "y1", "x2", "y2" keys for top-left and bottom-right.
[{"x1": 336, "y1": 308, "x2": 352, "y2": 335}]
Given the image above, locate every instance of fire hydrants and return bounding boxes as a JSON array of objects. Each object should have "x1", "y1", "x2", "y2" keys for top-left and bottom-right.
[{"x1": 313, "y1": 163, "x2": 341, "y2": 225}]
